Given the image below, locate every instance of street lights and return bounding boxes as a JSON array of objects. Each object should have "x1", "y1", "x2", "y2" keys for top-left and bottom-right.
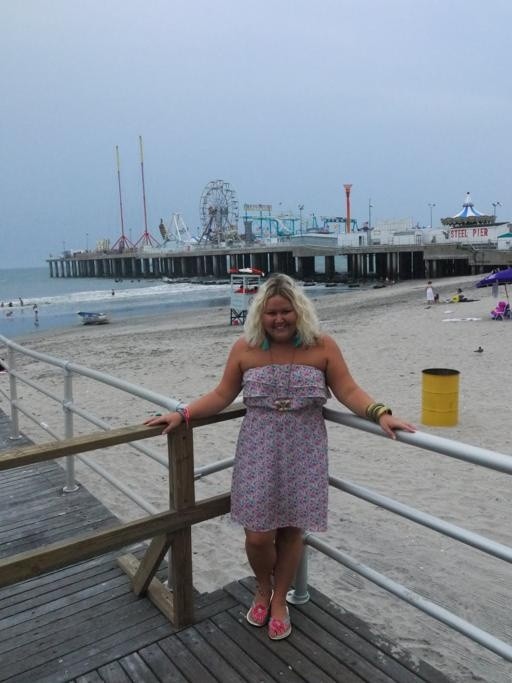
[
  {"x1": 427, "y1": 203, "x2": 435, "y2": 227},
  {"x1": 492, "y1": 201, "x2": 499, "y2": 217}
]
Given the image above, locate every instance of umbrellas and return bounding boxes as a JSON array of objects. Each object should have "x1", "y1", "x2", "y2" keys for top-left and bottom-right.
[{"x1": 475, "y1": 268, "x2": 512, "y2": 304}]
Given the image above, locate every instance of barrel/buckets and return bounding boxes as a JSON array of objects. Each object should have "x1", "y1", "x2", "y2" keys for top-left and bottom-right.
[{"x1": 420, "y1": 368, "x2": 461, "y2": 427}]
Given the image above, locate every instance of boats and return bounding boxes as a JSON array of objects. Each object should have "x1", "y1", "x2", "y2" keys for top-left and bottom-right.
[{"x1": 77, "y1": 311, "x2": 110, "y2": 324}]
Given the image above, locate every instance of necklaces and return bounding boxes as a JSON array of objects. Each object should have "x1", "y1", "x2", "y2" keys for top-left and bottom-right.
[{"x1": 265, "y1": 342, "x2": 298, "y2": 414}]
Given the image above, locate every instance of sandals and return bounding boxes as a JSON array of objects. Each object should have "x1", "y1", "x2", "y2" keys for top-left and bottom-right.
[{"x1": 245, "y1": 589, "x2": 292, "y2": 640}]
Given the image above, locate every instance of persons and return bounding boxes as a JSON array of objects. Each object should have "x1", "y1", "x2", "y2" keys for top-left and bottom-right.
[
  {"x1": 145, "y1": 274, "x2": 416, "y2": 642},
  {"x1": 448, "y1": 288, "x2": 464, "y2": 302},
  {"x1": 425, "y1": 282, "x2": 435, "y2": 306},
  {"x1": 33, "y1": 304, "x2": 38, "y2": 321}
]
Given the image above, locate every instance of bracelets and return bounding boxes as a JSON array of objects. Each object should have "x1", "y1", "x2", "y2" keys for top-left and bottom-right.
[
  {"x1": 177, "y1": 407, "x2": 192, "y2": 427},
  {"x1": 366, "y1": 402, "x2": 392, "y2": 424}
]
